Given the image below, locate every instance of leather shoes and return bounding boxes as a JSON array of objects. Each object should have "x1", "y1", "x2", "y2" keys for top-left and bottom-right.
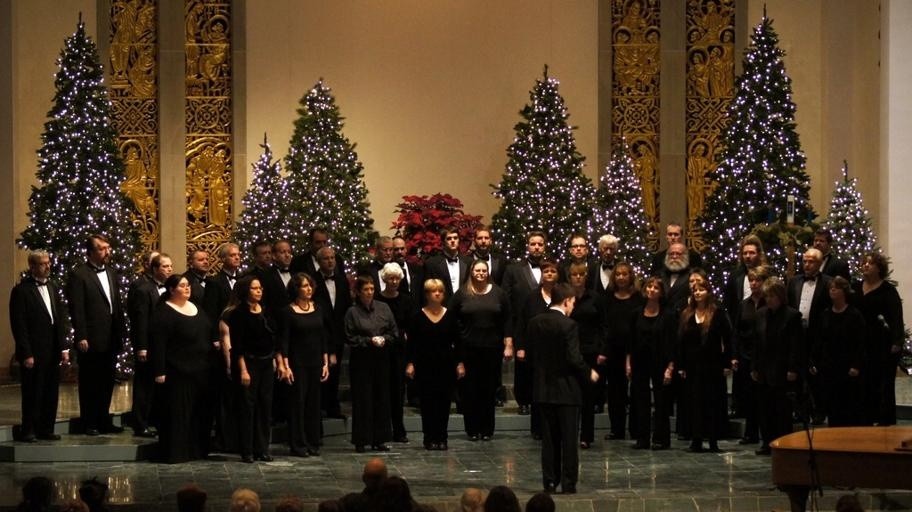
[
  {"x1": 542, "y1": 482, "x2": 556, "y2": 495},
  {"x1": 20, "y1": 422, "x2": 150, "y2": 444},
  {"x1": 634, "y1": 432, "x2": 771, "y2": 453},
  {"x1": 481, "y1": 434, "x2": 491, "y2": 441},
  {"x1": 393, "y1": 436, "x2": 409, "y2": 442},
  {"x1": 560, "y1": 480, "x2": 578, "y2": 495},
  {"x1": 424, "y1": 442, "x2": 448, "y2": 450},
  {"x1": 469, "y1": 433, "x2": 477, "y2": 440},
  {"x1": 518, "y1": 404, "x2": 529, "y2": 415}
]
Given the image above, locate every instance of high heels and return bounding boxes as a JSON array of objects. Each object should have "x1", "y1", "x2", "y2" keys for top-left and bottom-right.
[
  {"x1": 242, "y1": 456, "x2": 254, "y2": 462},
  {"x1": 253, "y1": 454, "x2": 275, "y2": 462},
  {"x1": 372, "y1": 443, "x2": 390, "y2": 451},
  {"x1": 354, "y1": 443, "x2": 366, "y2": 453}
]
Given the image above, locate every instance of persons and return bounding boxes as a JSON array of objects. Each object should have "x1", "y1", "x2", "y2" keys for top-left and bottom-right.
[
  {"x1": 109, "y1": 0, "x2": 158, "y2": 103},
  {"x1": 612, "y1": 2, "x2": 660, "y2": 87},
  {"x1": 685, "y1": 1, "x2": 738, "y2": 101},
  {"x1": 187, "y1": 146, "x2": 214, "y2": 219},
  {"x1": 119, "y1": 139, "x2": 158, "y2": 221},
  {"x1": 198, "y1": 15, "x2": 229, "y2": 89},
  {"x1": 148, "y1": 146, "x2": 160, "y2": 180},
  {"x1": 631, "y1": 137, "x2": 661, "y2": 255},
  {"x1": 208, "y1": 150, "x2": 230, "y2": 227},
  {"x1": 184, "y1": 2, "x2": 206, "y2": 87},
  {"x1": 687, "y1": 138, "x2": 719, "y2": 253}
]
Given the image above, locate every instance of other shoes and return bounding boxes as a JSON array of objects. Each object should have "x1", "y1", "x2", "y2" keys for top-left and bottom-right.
[
  {"x1": 812, "y1": 414, "x2": 826, "y2": 426},
  {"x1": 309, "y1": 447, "x2": 321, "y2": 456},
  {"x1": 580, "y1": 440, "x2": 591, "y2": 449},
  {"x1": 326, "y1": 412, "x2": 348, "y2": 419},
  {"x1": 604, "y1": 430, "x2": 625, "y2": 441}
]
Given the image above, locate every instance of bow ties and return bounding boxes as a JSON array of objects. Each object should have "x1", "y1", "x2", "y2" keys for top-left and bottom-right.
[
  {"x1": 36, "y1": 279, "x2": 49, "y2": 287},
  {"x1": 479, "y1": 256, "x2": 489, "y2": 261},
  {"x1": 531, "y1": 263, "x2": 541, "y2": 269},
  {"x1": 603, "y1": 264, "x2": 614, "y2": 271},
  {"x1": 802, "y1": 275, "x2": 816, "y2": 282},
  {"x1": 196, "y1": 275, "x2": 206, "y2": 283},
  {"x1": 227, "y1": 273, "x2": 237, "y2": 282},
  {"x1": 279, "y1": 257, "x2": 337, "y2": 282},
  {"x1": 448, "y1": 259, "x2": 458, "y2": 263},
  {"x1": 93, "y1": 267, "x2": 105, "y2": 273},
  {"x1": 156, "y1": 284, "x2": 165, "y2": 289}
]
